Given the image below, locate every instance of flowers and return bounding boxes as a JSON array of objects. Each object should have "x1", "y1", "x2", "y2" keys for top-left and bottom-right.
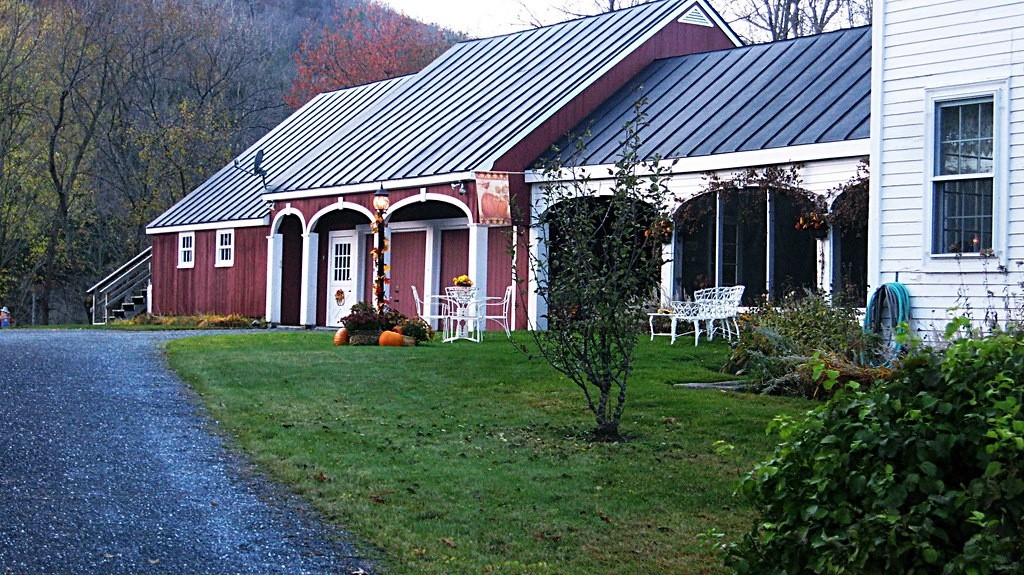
[{"x1": 453, "y1": 274, "x2": 473, "y2": 286}]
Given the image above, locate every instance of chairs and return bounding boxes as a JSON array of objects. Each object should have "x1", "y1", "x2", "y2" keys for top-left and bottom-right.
[
  {"x1": 670, "y1": 286, "x2": 745, "y2": 346},
  {"x1": 411, "y1": 285, "x2": 447, "y2": 340},
  {"x1": 480, "y1": 286, "x2": 511, "y2": 342},
  {"x1": 445, "y1": 287, "x2": 479, "y2": 344}
]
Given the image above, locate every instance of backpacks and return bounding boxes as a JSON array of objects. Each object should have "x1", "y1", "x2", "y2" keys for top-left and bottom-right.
[{"x1": 1, "y1": 318, "x2": 10, "y2": 327}]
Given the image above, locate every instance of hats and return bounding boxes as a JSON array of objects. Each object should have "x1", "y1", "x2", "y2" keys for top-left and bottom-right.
[{"x1": 0, "y1": 307, "x2": 10, "y2": 314}]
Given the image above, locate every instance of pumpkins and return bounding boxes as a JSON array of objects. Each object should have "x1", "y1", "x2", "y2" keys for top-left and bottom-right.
[
  {"x1": 378, "y1": 331, "x2": 402, "y2": 346},
  {"x1": 333, "y1": 328, "x2": 348, "y2": 345}
]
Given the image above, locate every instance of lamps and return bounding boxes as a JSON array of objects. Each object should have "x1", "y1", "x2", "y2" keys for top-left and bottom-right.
[{"x1": 451, "y1": 182, "x2": 466, "y2": 196}]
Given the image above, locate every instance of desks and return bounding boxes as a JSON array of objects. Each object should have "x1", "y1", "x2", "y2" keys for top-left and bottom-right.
[
  {"x1": 647, "y1": 312, "x2": 685, "y2": 342},
  {"x1": 430, "y1": 295, "x2": 501, "y2": 341}
]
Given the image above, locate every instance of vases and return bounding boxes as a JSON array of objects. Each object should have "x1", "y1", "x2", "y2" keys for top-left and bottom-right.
[{"x1": 456, "y1": 286, "x2": 471, "y2": 297}]
